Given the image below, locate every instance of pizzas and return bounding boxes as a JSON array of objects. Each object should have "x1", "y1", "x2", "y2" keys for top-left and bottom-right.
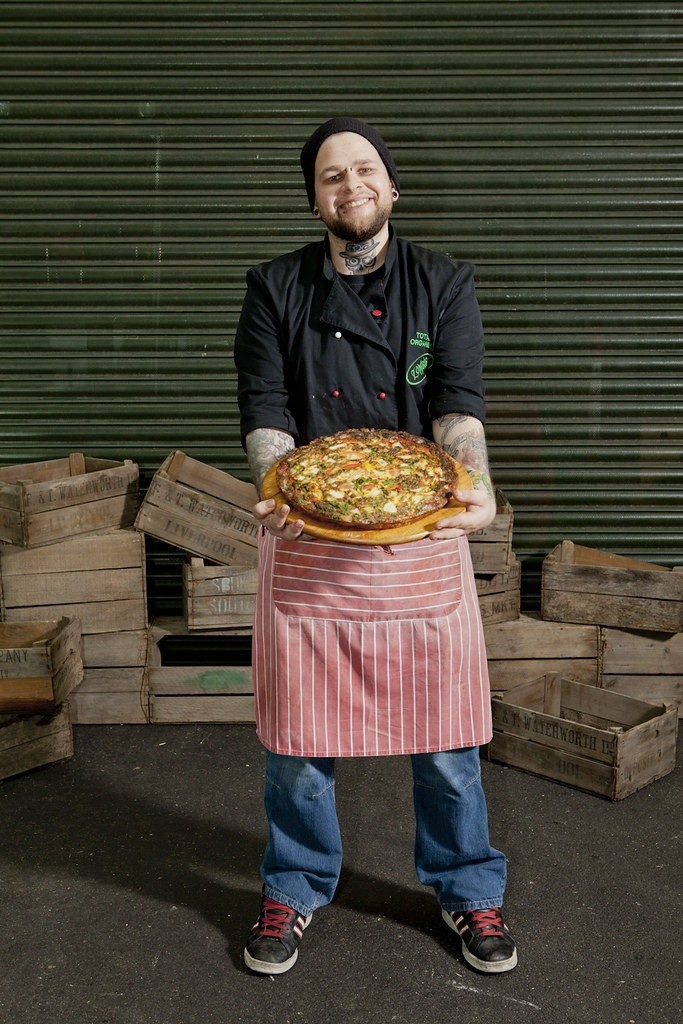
[{"x1": 276, "y1": 427, "x2": 459, "y2": 530}]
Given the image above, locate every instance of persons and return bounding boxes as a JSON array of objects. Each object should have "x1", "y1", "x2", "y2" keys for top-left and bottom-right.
[{"x1": 235, "y1": 114, "x2": 519, "y2": 975}]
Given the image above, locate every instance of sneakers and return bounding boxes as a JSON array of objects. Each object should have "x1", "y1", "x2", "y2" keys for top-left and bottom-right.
[
  {"x1": 243, "y1": 883, "x2": 313, "y2": 974},
  {"x1": 442, "y1": 908, "x2": 518, "y2": 973}
]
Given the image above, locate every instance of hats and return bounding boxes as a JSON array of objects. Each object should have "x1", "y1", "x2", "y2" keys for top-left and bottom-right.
[{"x1": 301, "y1": 118, "x2": 400, "y2": 221}]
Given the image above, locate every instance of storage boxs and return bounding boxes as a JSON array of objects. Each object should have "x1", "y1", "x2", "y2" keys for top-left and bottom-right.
[{"x1": 0, "y1": 452, "x2": 683, "y2": 802}]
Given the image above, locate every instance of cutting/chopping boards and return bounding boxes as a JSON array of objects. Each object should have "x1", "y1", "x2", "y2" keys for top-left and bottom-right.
[{"x1": 259, "y1": 454, "x2": 473, "y2": 546}]
